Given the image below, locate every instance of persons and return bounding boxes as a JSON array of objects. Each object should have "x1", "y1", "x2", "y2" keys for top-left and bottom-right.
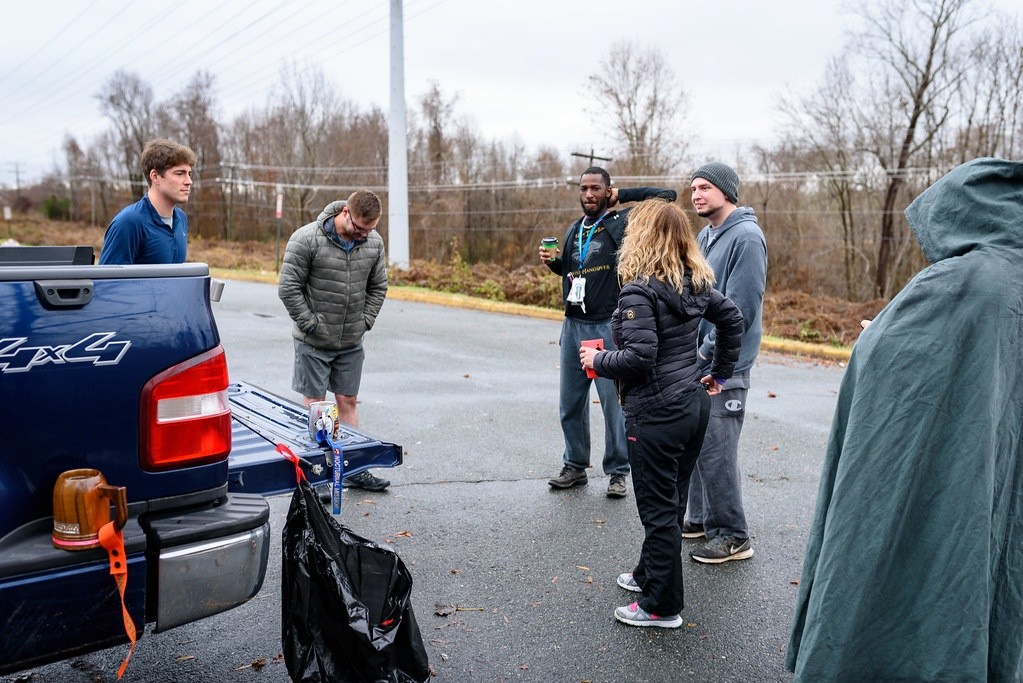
[
  {"x1": 579, "y1": 199, "x2": 745, "y2": 629},
  {"x1": 538, "y1": 166, "x2": 677, "y2": 496},
  {"x1": 97, "y1": 138, "x2": 198, "y2": 266},
  {"x1": 785, "y1": 159, "x2": 1023, "y2": 683},
  {"x1": 275, "y1": 189, "x2": 391, "y2": 503},
  {"x1": 682, "y1": 165, "x2": 768, "y2": 563}
]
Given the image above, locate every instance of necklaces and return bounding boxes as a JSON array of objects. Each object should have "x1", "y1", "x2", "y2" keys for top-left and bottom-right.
[{"x1": 582, "y1": 215, "x2": 602, "y2": 228}]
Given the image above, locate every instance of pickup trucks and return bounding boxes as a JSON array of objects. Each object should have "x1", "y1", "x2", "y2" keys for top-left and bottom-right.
[{"x1": 0, "y1": 244, "x2": 403, "y2": 678}]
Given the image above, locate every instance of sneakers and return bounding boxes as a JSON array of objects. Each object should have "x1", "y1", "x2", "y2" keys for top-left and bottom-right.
[
  {"x1": 614, "y1": 601, "x2": 683, "y2": 628},
  {"x1": 548, "y1": 465, "x2": 588, "y2": 487},
  {"x1": 342, "y1": 469, "x2": 391, "y2": 490},
  {"x1": 314, "y1": 484, "x2": 331, "y2": 503},
  {"x1": 606, "y1": 473, "x2": 627, "y2": 496},
  {"x1": 690, "y1": 536, "x2": 753, "y2": 564},
  {"x1": 617, "y1": 573, "x2": 643, "y2": 592},
  {"x1": 681, "y1": 520, "x2": 706, "y2": 538}
]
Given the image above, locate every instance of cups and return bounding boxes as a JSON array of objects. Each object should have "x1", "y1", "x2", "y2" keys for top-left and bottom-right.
[
  {"x1": 309, "y1": 400, "x2": 336, "y2": 441},
  {"x1": 541, "y1": 237, "x2": 559, "y2": 262},
  {"x1": 581, "y1": 339, "x2": 604, "y2": 379},
  {"x1": 51, "y1": 468, "x2": 128, "y2": 551}
]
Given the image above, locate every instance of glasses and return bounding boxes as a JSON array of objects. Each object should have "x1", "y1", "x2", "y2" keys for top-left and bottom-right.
[{"x1": 347, "y1": 210, "x2": 377, "y2": 234}]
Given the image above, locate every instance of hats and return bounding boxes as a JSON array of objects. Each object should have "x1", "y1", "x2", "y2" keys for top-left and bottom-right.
[{"x1": 691, "y1": 161, "x2": 740, "y2": 205}]
